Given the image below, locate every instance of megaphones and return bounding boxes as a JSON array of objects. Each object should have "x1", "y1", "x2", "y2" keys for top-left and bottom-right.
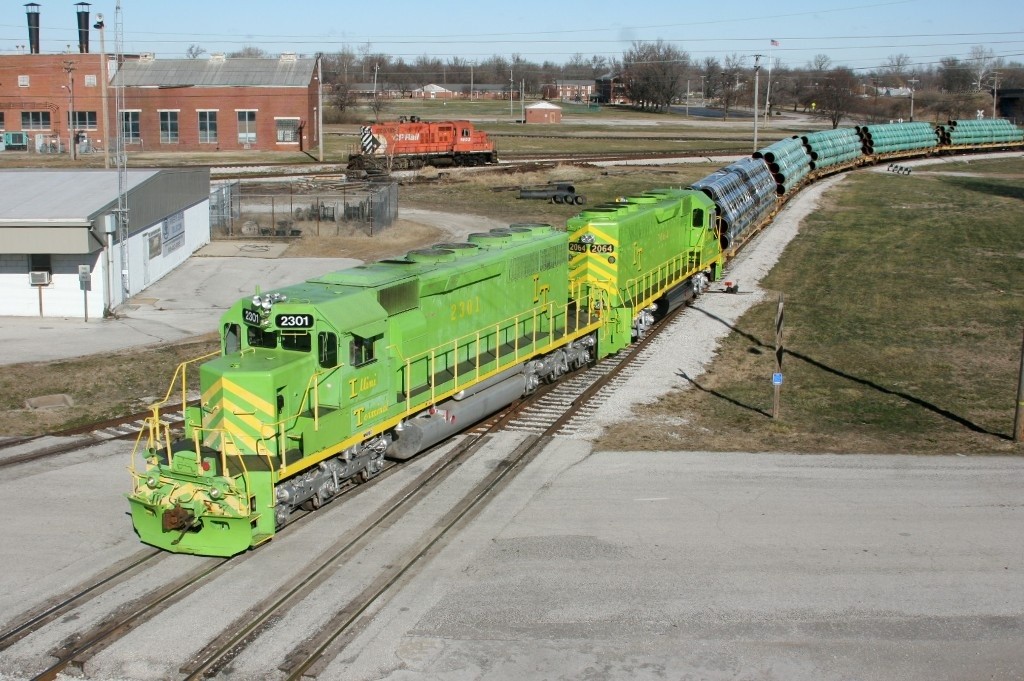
[{"x1": 95, "y1": 13, "x2": 103, "y2": 22}]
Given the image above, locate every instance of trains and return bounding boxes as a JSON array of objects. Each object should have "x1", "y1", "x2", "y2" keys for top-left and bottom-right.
[
  {"x1": 123, "y1": 115, "x2": 1023, "y2": 562},
  {"x1": 345, "y1": 114, "x2": 498, "y2": 177}
]
